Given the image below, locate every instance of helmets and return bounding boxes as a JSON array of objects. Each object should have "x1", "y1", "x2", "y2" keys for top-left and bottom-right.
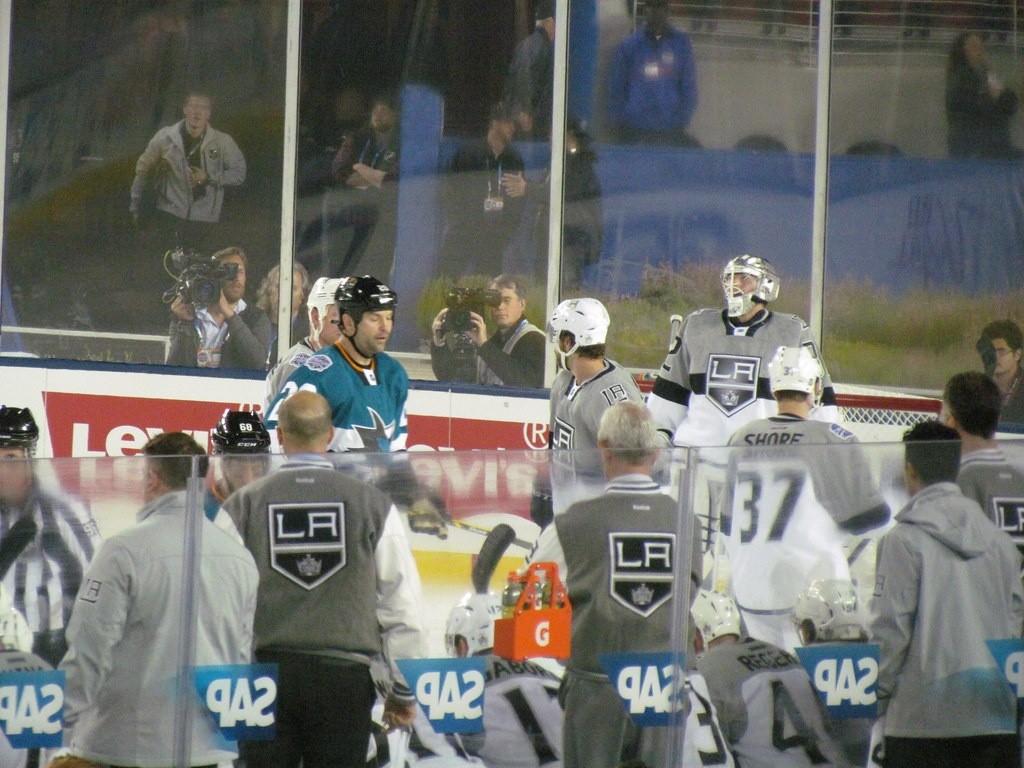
[
  {"x1": 209, "y1": 408, "x2": 270, "y2": 453},
  {"x1": 0, "y1": 406, "x2": 39, "y2": 448},
  {"x1": 721, "y1": 254, "x2": 781, "y2": 317},
  {"x1": 691, "y1": 589, "x2": 741, "y2": 645},
  {"x1": 546, "y1": 298, "x2": 610, "y2": 346},
  {"x1": 444, "y1": 594, "x2": 503, "y2": 653},
  {"x1": 306, "y1": 276, "x2": 349, "y2": 320},
  {"x1": 334, "y1": 275, "x2": 398, "y2": 325},
  {"x1": 792, "y1": 579, "x2": 862, "y2": 639},
  {"x1": 0, "y1": 608, "x2": 33, "y2": 653},
  {"x1": 768, "y1": 345, "x2": 824, "y2": 400}
]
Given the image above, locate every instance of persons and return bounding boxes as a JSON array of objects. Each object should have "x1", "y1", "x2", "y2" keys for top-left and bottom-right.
[
  {"x1": 129, "y1": 92, "x2": 246, "y2": 255},
  {"x1": 0, "y1": 606, "x2": 55, "y2": 768},
  {"x1": 607, "y1": 0, "x2": 698, "y2": 146},
  {"x1": 165, "y1": 249, "x2": 452, "y2": 539},
  {"x1": 939, "y1": 372, "x2": 1024, "y2": 575},
  {"x1": 43, "y1": 431, "x2": 261, "y2": 768},
  {"x1": 646, "y1": 256, "x2": 840, "y2": 589},
  {"x1": 440, "y1": 0, "x2": 603, "y2": 294},
  {"x1": 519, "y1": 400, "x2": 703, "y2": 768},
  {"x1": 0, "y1": 405, "x2": 103, "y2": 669},
  {"x1": 295, "y1": 103, "x2": 401, "y2": 275},
  {"x1": 210, "y1": 390, "x2": 423, "y2": 768},
  {"x1": 690, "y1": 590, "x2": 849, "y2": 768},
  {"x1": 443, "y1": 591, "x2": 572, "y2": 768},
  {"x1": 977, "y1": 320, "x2": 1024, "y2": 434},
  {"x1": 427, "y1": 273, "x2": 546, "y2": 390},
  {"x1": 713, "y1": 345, "x2": 892, "y2": 658},
  {"x1": 945, "y1": 32, "x2": 1017, "y2": 154},
  {"x1": 204, "y1": 408, "x2": 271, "y2": 522},
  {"x1": 529, "y1": 298, "x2": 645, "y2": 532},
  {"x1": 868, "y1": 421, "x2": 1024, "y2": 768}
]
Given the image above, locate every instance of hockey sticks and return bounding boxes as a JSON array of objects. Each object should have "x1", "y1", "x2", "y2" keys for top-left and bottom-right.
[
  {"x1": 0, "y1": 517, "x2": 39, "y2": 583},
  {"x1": 847, "y1": 460, "x2": 881, "y2": 566},
  {"x1": 668, "y1": 311, "x2": 683, "y2": 349},
  {"x1": 473, "y1": 521, "x2": 517, "y2": 591},
  {"x1": 393, "y1": 503, "x2": 533, "y2": 550}
]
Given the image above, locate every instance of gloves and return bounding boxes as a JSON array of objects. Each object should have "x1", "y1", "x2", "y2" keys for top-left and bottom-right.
[{"x1": 406, "y1": 495, "x2": 448, "y2": 540}]
